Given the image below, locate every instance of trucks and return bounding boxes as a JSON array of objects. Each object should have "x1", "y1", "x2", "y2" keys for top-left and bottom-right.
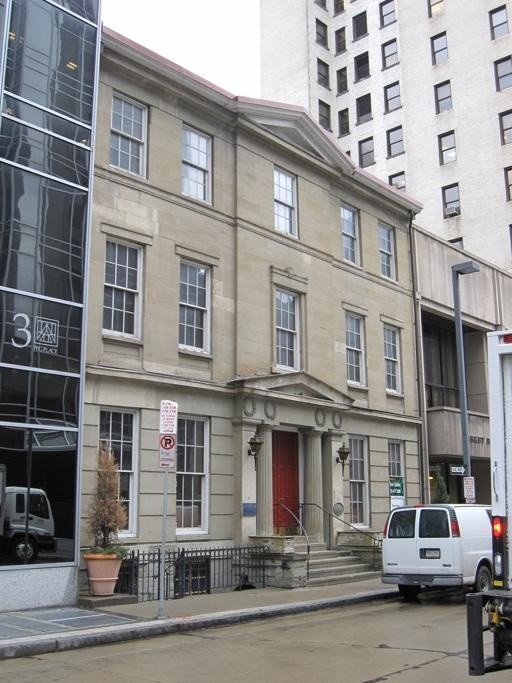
[{"x1": 1, "y1": 462, "x2": 58, "y2": 562}]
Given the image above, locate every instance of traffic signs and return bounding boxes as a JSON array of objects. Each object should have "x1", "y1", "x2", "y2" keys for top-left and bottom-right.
[{"x1": 449, "y1": 464, "x2": 469, "y2": 476}]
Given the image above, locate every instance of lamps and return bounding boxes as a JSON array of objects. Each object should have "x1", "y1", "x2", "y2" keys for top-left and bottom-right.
[
  {"x1": 336, "y1": 441, "x2": 351, "y2": 465},
  {"x1": 248, "y1": 431, "x2": 264, "y2": 457}
]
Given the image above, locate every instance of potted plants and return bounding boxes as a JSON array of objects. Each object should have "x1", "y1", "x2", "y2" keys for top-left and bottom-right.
[{"x1": 82, "y1": 439, "x2": 129, "y2": 598}]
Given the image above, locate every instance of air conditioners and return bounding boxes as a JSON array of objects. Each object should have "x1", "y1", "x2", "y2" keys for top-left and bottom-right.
[{"x1": 445, "y1": 206, "x2": 458, "y2": 216}]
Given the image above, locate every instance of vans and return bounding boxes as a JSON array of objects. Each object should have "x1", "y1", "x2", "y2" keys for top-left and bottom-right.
[{"x1": 381, "y1": 505, "x2": 493, "y2": 599}]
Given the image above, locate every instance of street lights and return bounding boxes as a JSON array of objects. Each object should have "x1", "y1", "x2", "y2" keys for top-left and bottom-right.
[{"x1": 452, "y1": 261, "x2": 480, "y2": 477}]
